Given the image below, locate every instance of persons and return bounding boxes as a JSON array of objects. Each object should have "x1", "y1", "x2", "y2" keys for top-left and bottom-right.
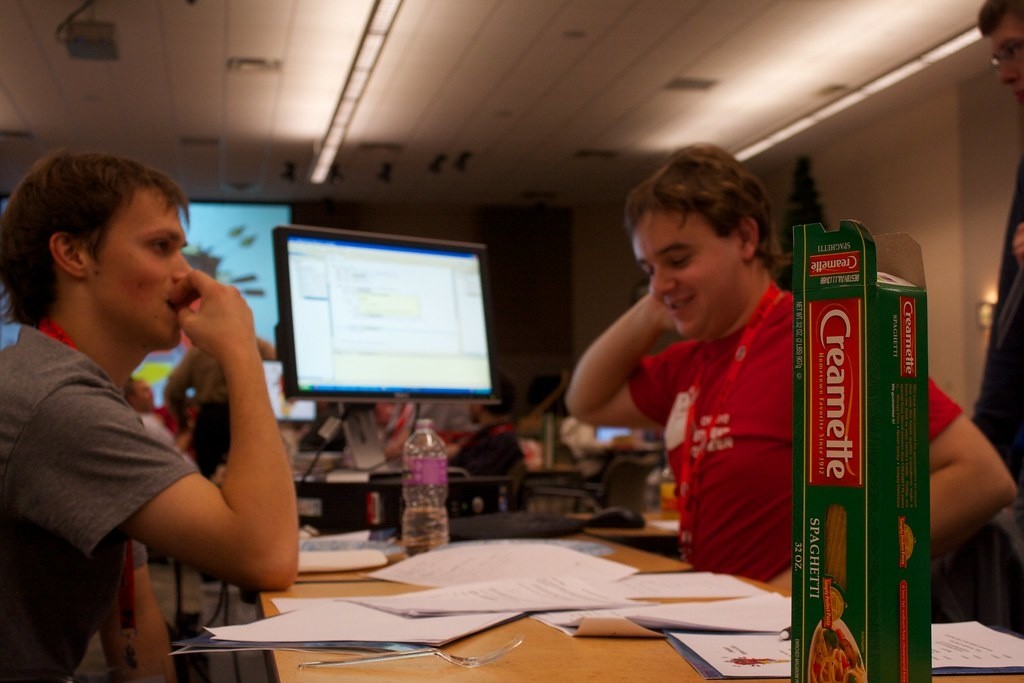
[
  {"x1": 166, "y1": 336, "x2": 279, "y2": 485},
  {"x1": 122, "y1": 378, "x2": 195, "y2": 461},
  {"x1": 975, "y1": 0, "x2": 1024, "y2": 495},
  {"x1": 563, "y1": 415, "x2": 613, "y2": 483},
  {"x1": 564, "y1": 142, "x2": 1017, "y2": 593},
  {"x1": 446, "y1": 372, "x2": 524, "y2": 521},
  {"x1": 1, "y1": 149, "x2": 300, "y2": 683}
]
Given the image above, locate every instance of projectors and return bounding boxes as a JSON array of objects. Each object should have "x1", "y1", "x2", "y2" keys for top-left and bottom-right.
[{"x1": 65, "y1": 20, "x2": 118, "y2": 62}]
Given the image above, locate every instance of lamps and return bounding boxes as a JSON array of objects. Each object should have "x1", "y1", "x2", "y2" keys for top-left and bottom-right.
[
  {"x1": 306, "y1": 0, "x2": 402, "y2": 186},
  {"x1": 731, "y1": 25, "x2": 983, "y2": 167}
]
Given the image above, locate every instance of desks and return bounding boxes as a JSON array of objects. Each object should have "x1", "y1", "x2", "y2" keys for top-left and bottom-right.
[
  {"x1": 295, "y1": 472, "x2": 512, "y2": 530},
  {"x1": 255, "y1": 536, "x2": 1023, "y2": 682}
]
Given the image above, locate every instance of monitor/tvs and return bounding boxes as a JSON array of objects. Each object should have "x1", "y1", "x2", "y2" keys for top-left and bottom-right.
[
  {"x1": 595, "y1": 427, "x2": 631, "y2": 443},
  {"x1": 271, "y1": 223, "x2": 504, "y2": 406}
]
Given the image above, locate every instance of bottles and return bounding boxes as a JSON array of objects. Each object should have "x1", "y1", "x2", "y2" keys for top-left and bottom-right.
[
  {"x1": 658, "y1": 463, "x2": 680, "y2": 518},
  {"x1": 402, "y1": 417, "x2": 449, "y2": 557}
]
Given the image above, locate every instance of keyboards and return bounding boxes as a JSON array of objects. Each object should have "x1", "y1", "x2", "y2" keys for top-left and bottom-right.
[{"x1": 447, "y1": 507, "x2": 586, "y2": 542}]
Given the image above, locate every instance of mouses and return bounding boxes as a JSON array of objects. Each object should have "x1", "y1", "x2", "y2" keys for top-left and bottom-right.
[{"x1": 584, "y1": 504, "x2": 645, "y2": 529}]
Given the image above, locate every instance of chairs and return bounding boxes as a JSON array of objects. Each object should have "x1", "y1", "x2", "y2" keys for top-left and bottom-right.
[
  {"x1": 584, "y1": 453, "x2": 660, "y2": 512},
  {"x1": 516, "y1": 468, "x2": 583, "y2": 514}
]
